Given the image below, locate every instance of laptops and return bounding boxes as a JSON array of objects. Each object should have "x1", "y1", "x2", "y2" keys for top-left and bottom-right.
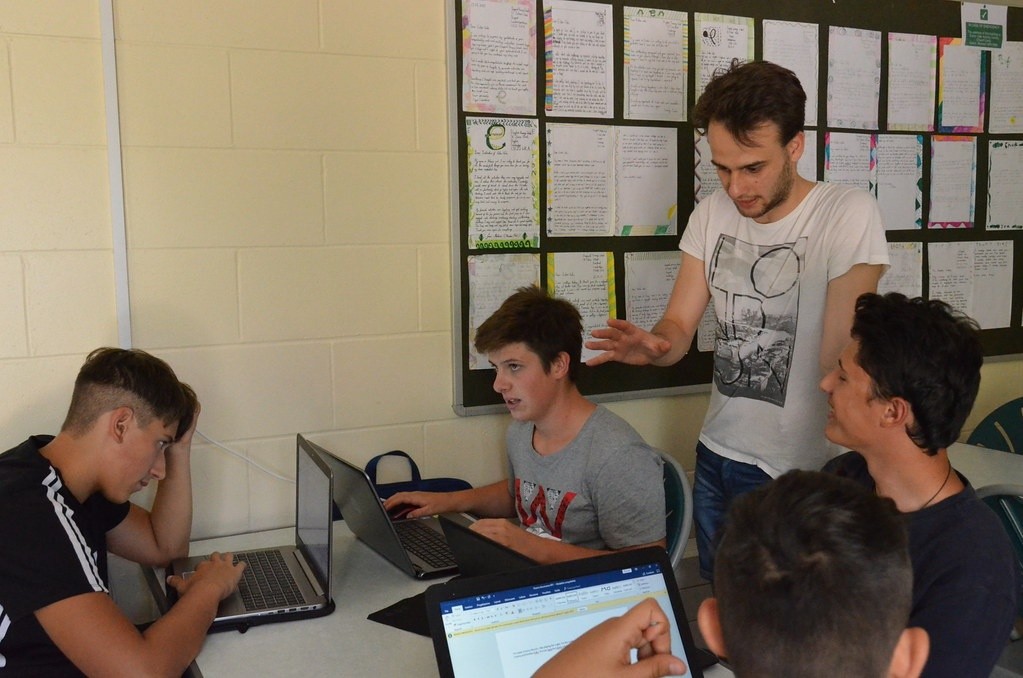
[
  {"x1": 422, "y1": 544, "x2": 706, "y2": 678},
  {"x1": 437, "y1": 513, "x2": 719, "y2": 669},
  {"x1": 170, "y1": 433, "x2": 336, "y2": 623},
  {"x1": 306, "y1": 439, "x2": 460, "y2": 579}
]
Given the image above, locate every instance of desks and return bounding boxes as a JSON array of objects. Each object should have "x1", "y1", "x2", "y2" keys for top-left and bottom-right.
[{"x1": 140, "y1": 520, "x2": 461, "y2": 678}]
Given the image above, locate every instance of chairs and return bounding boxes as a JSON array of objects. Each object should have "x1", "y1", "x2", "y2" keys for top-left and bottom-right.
[
  {"x1": 663, "y1": 452, "x2": 693, "y2": 570},
  {"x1": 966, "y1": 395, "x2": 1023, "y2": 456}
]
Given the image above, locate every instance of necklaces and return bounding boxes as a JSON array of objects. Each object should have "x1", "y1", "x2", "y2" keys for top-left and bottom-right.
[{"x1": 921, "y1": 458, "x2": 952, "y2": 510}]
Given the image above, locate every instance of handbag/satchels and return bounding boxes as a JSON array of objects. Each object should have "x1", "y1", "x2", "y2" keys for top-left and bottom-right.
[{"x1": 333, "y1": 450, "x2": 474, "y2": 519}]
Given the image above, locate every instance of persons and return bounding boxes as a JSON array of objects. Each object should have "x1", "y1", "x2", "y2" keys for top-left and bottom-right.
[
  {"x1": 0, "y1": 348, "x2": 249, "y2": 678},
  {"x1": 585, "y1": 61, "x2": 893, "y2": 599},
  {"x1": 382, "y1": 289, "x2": 667, "y2": 565},
  {"x1": 531, "y1": 293, "x2": 1013, "y2": 678}
]
